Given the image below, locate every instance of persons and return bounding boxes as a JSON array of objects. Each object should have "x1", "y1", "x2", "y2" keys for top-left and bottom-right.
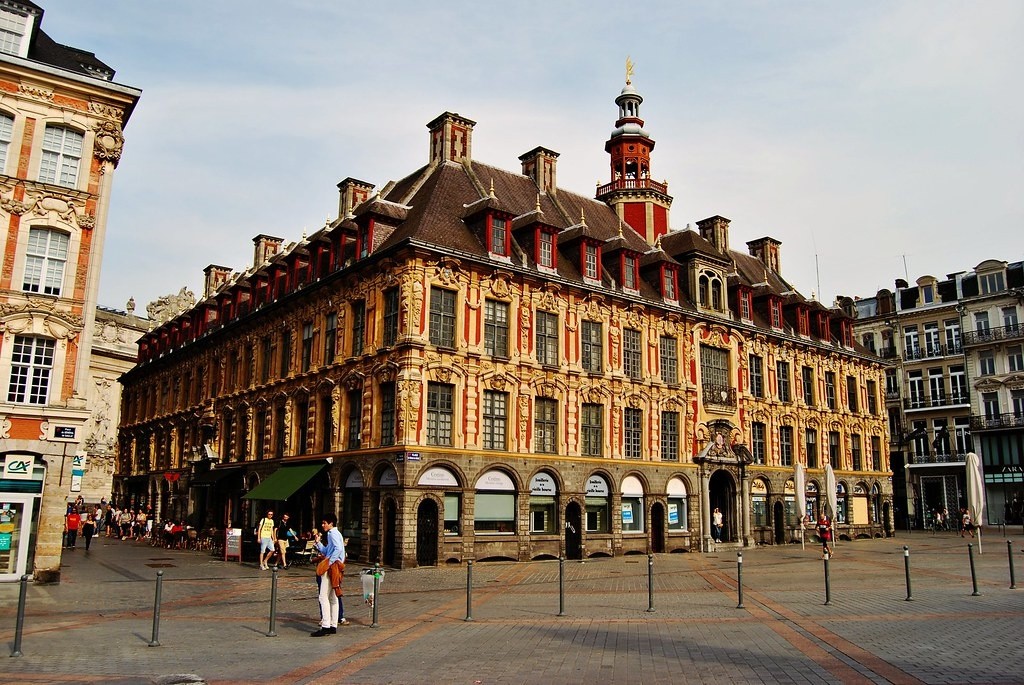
[
  {"x1": 309, "y1": 532, "x2": 350, "y2": 626},
  {"x1": 310, "y1": 512, "x2": 346, "y2": 637},
  {"x1": 64, "y1": 494, "x2": 226, "y2": 550},
  {"x1": 815, "y1": 513, "x2": 833, "y2": 560},
  {"x1": 926, "y1": 489, "x2": 1022, "y2": 537},
  {"x1": 712, "y1": 506, "x2": 723, "y2": 543},
  {"x1": 274, "y1": 512, "x2": 298, "y2": 571},
  {"x1": 258, "y1": 509, "x2": 275, "y2": 571}
]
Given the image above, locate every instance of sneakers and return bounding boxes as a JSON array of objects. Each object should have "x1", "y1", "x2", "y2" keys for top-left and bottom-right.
[
  {"x1": 263, "y1": 563, "x2": 269, "y2": 569},
  {"x1": 259, "y1": 565, "x2": 266, "y2": 570}
]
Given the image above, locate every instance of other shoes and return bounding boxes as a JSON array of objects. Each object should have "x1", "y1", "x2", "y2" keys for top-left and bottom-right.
[
  {"x1": 326, "y1": 627, "x2": 337, "y2": 634},
  {"x1": 310, "y1": 627, "x2": 330, "y2": 637}
]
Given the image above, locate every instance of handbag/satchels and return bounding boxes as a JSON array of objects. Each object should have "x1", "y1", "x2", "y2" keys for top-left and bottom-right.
[{"x1": 253, "y1": 525, "x2": 261, "y2": 536}]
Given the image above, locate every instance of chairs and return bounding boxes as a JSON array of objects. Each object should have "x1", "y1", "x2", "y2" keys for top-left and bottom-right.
[{"x1": 288, "y1": 533, "x2": 350, "y2": 567}]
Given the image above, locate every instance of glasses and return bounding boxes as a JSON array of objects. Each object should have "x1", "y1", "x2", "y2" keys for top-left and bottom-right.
[{"x1": 268, "y1": 514, "x2": 273, "y2": 516}]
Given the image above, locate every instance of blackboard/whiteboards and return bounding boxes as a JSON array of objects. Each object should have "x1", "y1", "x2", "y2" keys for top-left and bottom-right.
[{"x1": 225, "y1": 528, "x2": 241, "y2": 556}]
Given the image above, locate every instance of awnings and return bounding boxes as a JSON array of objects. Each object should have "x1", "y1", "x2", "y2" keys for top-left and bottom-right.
[
  {"x1": 186, "y1": 467, "x2": 243, "y2": 490},
  {"x1": 240, "y1": 462, "x2": 329, "y2": 501}
]
